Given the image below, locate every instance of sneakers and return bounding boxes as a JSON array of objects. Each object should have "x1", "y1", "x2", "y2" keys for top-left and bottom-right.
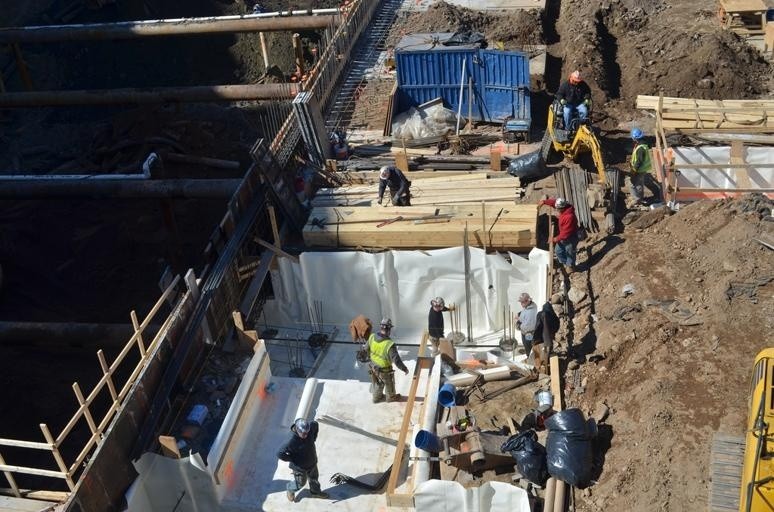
[
  {"x1": 311, "y1": 493, "x2": 328, "y2": 498},
  {"x1": 287, "y1": 490, "x2": 295, "y2": 501},
  {"x1": 373, "y1": 394, "x2": 386, "y2": 402},
  {"x1": 386, "y1": 394, "x2": 400, "y2": 401}
]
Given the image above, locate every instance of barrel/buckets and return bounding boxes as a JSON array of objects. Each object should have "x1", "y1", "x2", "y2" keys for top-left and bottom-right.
[
  {"x1": 413, "y1": 429, "x2": 443, "y2": 456},
  {"x1": 438, "y1": 383, "x2": 457, "y2": 408},
  {"x1": 537, "y1": 391, "x2": 553, "y2": 407}
]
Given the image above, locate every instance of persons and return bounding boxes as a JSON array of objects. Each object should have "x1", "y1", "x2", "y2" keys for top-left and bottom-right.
[
  {"x1": 361, "y1": 317, "x2": 409, "y2": 402},
  {"x1": 628, "y1": 127, "x2": 661, "y2": 207},
  {"x1": 428, "y1": 297, "x2": 453, "y2": 359},
  {"x1": 377, "y1": 165, "x2": 411, "y2": 207},
  {"x1": 514, "y1": 292, "x2": 539, "y2": 356},
  {"x1": 556, "y1": 71, "x2": 592, "y2": 134},
  {"x1": 538, "y1": 197, "x2": 580, "y2": 276},
  {"x1": 274, "y1": 419, "x2": 329, "y2": 502}
]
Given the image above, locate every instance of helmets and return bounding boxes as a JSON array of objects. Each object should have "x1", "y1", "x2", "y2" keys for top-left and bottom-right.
[
  {"x1": 517, "y1": 293, "x2": 533, "y2": 302},
  {"x1": 380, "y1": 317, "x2": 395, "y2": 327},
  {"x1": 379, "y1": 166, "x2": 390, "y2": 180},
  {"x1": 555, "y1": 198, "x2": 566, "y2": 208},
  {"x1": 570, "y1": 70, "x2": 582, "y2": 83},
  {"x1": 431, "y1": 297, "x2": 445, "y2": 307},
  {"x1": 631, "y1": 128, "x2": 643, "y2": 139},
  {"x1": 296, "y1": 418, "x2": 311, "y2": 433}
]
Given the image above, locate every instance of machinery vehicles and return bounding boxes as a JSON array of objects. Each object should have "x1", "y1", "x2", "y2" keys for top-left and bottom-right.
[
  {"x1": 706, "y1": 347, "x2": 774, "y2": 512},
  {"x1": 541, "y1": 97, "x2": 614, "y2": 211}
]
